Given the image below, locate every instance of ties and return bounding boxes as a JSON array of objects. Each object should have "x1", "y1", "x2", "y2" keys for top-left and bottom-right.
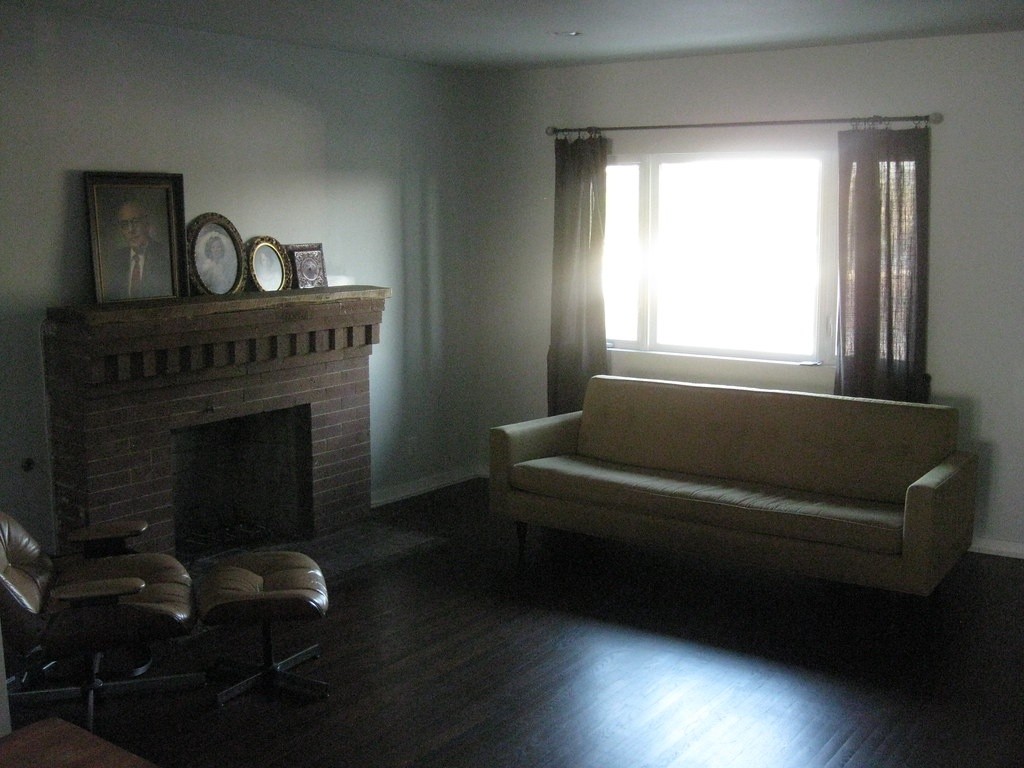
[{"x1": 130, "y1": 255, "x2": 142, "y2": 298}]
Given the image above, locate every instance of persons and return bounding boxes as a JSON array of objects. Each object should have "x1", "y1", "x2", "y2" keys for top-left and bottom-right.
[
  {"x1": 111, "y1": 198, "x2": 173, "y2": 299},
  {"x1": 201, "y1": 234, "x2": 229, "y2": 293},
  {"x1": 256, "y1": 251, "x2": 280, "y2": 290}
]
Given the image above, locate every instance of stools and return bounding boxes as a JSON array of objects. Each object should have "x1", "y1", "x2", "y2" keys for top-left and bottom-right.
[{"x1": 193, "y1": 551, "x2": 330, "y2": 707}]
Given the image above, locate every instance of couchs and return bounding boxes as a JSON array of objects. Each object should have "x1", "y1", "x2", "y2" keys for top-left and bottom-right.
[{"x1": 488, "y1": 374, "x2": 979, "y2": 624}]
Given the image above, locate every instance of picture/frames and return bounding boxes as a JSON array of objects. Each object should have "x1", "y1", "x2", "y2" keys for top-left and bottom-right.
[
  {"x1": 84, "y1": 171, "x2": 190, "y2": 303},
  {"x1": 186, "y1": 212, "x2": 248, "y2": 295},
  {"x1": 249, "y1": 236, "x2": 292, "y2": 292},
  {"x1": 287, "y1": 242, "x2": 329, "y2": 288}
]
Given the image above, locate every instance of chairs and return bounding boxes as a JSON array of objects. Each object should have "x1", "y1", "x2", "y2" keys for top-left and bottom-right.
[{"x1": 0, "y1": 509, "x2": 205, "y2": 735}]
